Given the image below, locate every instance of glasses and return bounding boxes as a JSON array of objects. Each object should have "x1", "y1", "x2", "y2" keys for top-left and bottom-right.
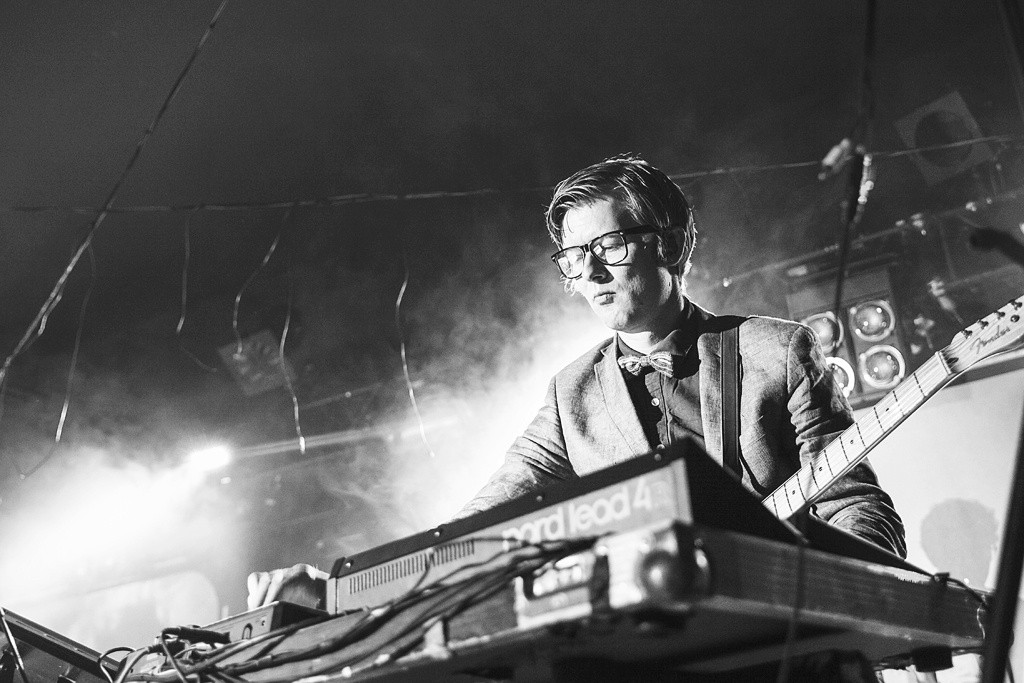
[{"x1": 550, "y1": 224, "x2": 662, "y2": 279}]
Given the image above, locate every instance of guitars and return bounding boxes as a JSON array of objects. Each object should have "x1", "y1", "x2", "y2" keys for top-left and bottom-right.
[{"x1": 766, "y1": 293, "x2": 1024, "y2": 520}]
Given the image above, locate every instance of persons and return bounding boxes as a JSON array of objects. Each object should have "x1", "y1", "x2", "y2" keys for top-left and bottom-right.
[{"x1": 247, "y1": 159, "x2": 908, "y2": 610}]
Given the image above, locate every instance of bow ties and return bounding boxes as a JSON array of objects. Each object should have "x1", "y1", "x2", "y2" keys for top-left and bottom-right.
[{"x1": 617, "y1": 350, "x2": 674, "y2": 380}]
